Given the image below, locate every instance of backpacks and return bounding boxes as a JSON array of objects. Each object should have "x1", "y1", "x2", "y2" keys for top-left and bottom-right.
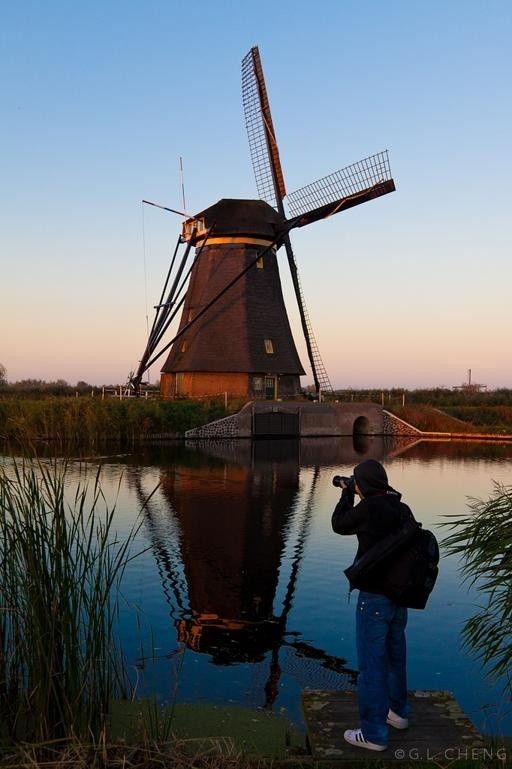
[{"x1": 384, "y1": 524, "x2": 442, "y2": 613}]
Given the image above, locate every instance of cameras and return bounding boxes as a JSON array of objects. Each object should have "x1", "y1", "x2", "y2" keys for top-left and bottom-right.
[{"x1": 332, "y1": 475, "x2": 359, "y2": 494}]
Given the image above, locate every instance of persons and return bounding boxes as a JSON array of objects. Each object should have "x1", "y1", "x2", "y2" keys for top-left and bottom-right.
[{"x1": 331, "y1": 458, "x2": 441, "y2": 750}]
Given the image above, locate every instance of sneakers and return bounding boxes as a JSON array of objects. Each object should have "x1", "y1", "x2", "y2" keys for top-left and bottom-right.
[
  {"x1": 385, "y1": 707, "x2": 410, "y2": 729},
  {"x1": 342, "y1": 726, "x2": 387, "y2": 753}
]
